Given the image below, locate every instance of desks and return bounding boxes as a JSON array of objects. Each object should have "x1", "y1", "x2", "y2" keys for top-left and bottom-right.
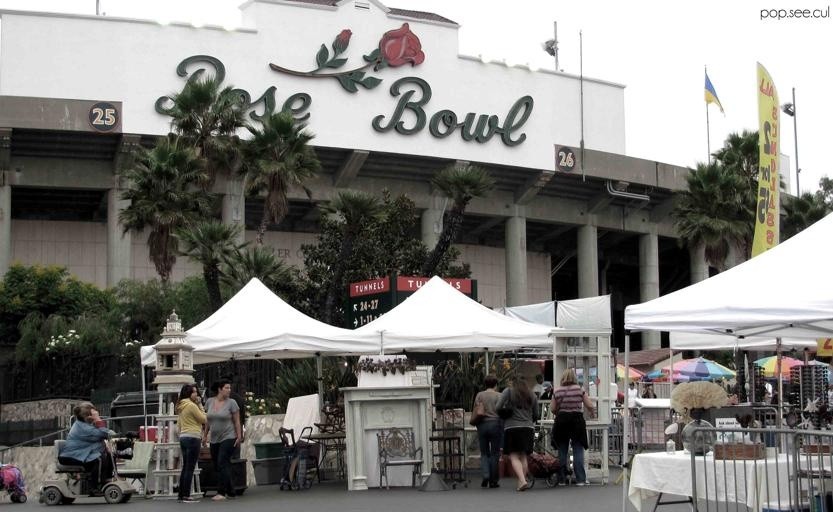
[{"x1": 623, "y1": 444, "x2": 831, "y2": 511}]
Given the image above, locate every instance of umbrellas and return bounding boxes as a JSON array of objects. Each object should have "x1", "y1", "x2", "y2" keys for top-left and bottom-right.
[{"x1": 573, "y1": 356, "x2": 829, "y2": 382}]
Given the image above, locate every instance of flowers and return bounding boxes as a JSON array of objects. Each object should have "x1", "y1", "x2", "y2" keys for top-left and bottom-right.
[{"x1": 670, "y1": 380, "x2": 729, "y2": 420}]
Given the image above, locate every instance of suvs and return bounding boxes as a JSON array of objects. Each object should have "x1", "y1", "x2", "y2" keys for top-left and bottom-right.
[
  {"x1": 761, "y1": 377, "x2": 793, "y2": 417},
  {"x1": 113, "y1": 388, "x2": 214, "y2": 439},
  {"x1": 477, "y1": 347, "x2": 554, "y2": 400}
]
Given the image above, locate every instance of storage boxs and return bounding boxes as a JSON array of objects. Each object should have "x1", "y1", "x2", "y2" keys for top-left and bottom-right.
[
  {"x1": 253, "y1": 442, "x2": 285, "y2": 458},
  {"x1": 250, "y1": 459, "x2": 285, "y2": 486}
]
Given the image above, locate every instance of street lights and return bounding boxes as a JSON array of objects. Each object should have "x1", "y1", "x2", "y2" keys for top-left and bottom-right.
[
  {"x1": 540, "y1": 20, "x2": 563, "y2": 73},
  {"x1": 782, "y1": 86, "x2": 809, "y2": 198}
]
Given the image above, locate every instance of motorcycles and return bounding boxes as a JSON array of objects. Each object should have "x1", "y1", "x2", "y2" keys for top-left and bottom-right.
[{"x1": 38, "y1": 419, "x2": 135, "y2": 503}]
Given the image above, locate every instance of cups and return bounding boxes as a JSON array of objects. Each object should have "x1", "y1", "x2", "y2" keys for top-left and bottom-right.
[
  {"x1": 666, "y1": 442, "x2": 675, "y2": 455},
  {"x1": 766, "y1": 447, "x2": 778, "y2": 458}
]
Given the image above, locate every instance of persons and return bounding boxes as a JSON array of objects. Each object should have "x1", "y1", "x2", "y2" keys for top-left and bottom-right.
[
  {"x1": 764, "y1": 379, "x2": 773, "y2": 406},
  {"x1": 59, "y1": 403, "x2": 116, "y2": 497},
  {"x1": 202, "y1": 380, "x2": 242, "y2": 501},
  {"x1": 177, "y1": 385, "x2": 207, "y2": 503},
  {"x1": 472, "y1": 370, "x2": 657, "y2": 491}
]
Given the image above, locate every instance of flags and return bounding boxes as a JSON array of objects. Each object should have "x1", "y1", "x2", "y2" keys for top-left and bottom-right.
[{"x1": 704, "y1": 75, "x2": 725, "y2": 111}]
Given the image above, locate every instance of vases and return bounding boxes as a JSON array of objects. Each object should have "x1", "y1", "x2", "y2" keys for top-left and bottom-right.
[{"x1": 681, "y1": 417, "x2": 717, "y2": 456}]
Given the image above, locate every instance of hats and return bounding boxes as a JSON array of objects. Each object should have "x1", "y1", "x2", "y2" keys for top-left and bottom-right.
[{"x1": 542, "y1": 381, "x2": 552, "y2": 390}]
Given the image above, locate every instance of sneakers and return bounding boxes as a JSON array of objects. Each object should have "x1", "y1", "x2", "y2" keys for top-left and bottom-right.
[
  {"x1": 480, "y1": 478, "x2": 528, "y2": 491},
  {"x1": 176, "y1": 494, "x2": 237, "y2": 503},
  {"x1": 576, "y1": 480, "x2": 591, "y2": 487}
]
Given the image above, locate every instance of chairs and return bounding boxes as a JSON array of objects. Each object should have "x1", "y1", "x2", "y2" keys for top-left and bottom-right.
[
  {"x1": 54, "y1": 439, "x2": 87, "y2": 474},
  {"x1": 274, "y1": 408, "x2": 346, "y2": 493},
  {"x1": 115, "y1": 441, "x2": 156, "y2": 500},
  {"x1": 378, "y1": 426, "x2": 424, "y2": 491}
]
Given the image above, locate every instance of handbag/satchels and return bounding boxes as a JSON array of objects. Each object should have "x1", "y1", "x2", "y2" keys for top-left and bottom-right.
[
  {"x1": 469, "y1": 401, "x2": 486, "y2": 426},
  {"x1": 114, "y1": 436, "x2": 134, "y2": 460},
  {"x1": 498, "y1": 398, "x2": 515, "y2": 421}
]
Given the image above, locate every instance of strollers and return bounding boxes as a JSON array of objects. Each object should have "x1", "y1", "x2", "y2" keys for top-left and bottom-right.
[
  {"x1": 511, "y1": 425, "x2": 574, "y2": 490},
  {"x1": 278, "y1": 426, "x2": 323, "y2": 492},
  {"x1": 0, "y1": 463, "x2": 27, "y2": 503}
]
